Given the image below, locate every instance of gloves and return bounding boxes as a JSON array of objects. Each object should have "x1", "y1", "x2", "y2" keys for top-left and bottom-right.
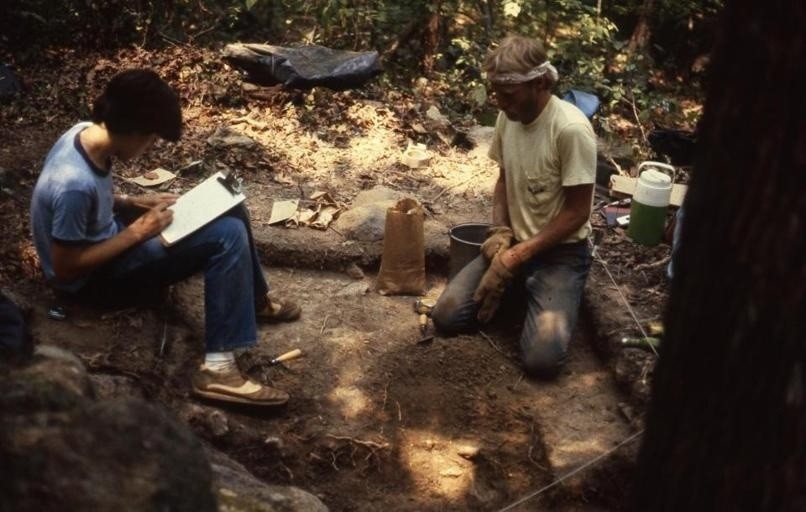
[
  {"x1": 473, "y1": 252, "x2": 515, "y2": 326},
  {"x1": 480, "y1": 225, "x2": 513, "y2": 262}
]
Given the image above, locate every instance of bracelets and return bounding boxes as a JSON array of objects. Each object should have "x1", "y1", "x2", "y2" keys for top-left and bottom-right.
[{"x1": 119, "y1": 193, "x2": 128, "y2": 208}]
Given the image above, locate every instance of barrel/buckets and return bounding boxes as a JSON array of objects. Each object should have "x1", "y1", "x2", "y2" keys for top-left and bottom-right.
[{"x1": 448, "y1": 222, "x2": 498, "y2": 280}]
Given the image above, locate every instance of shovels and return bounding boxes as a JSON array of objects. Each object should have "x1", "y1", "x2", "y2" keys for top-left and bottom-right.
[{"x1": 245, "y1": 348, "x2": 301, "y2": 373}]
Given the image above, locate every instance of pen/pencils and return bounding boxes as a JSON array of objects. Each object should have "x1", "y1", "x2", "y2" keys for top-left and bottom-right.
[{"x1": 133, "y1": 202, "x2": 153, "y2": 209}]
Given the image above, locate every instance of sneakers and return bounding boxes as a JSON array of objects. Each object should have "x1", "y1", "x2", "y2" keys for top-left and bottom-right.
[
  {"x1": 254, "y1": 294, "x2": 302, "y2": 320},
  {"x1": 192, "y1": 366, "x2": 289, "y2": 407}
]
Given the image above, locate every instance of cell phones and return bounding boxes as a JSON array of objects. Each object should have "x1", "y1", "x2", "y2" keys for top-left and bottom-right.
[{"x1": 50, "y1": 303, "x2": 66, "y2": 320}]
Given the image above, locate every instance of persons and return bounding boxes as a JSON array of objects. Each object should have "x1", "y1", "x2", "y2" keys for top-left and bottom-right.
[
  {"x1": 431, "y1": 38, "x2": 597, "y2": 379},
  {"x1": 30, "y1": 69, "x2": 301, "y2": 408}
]
